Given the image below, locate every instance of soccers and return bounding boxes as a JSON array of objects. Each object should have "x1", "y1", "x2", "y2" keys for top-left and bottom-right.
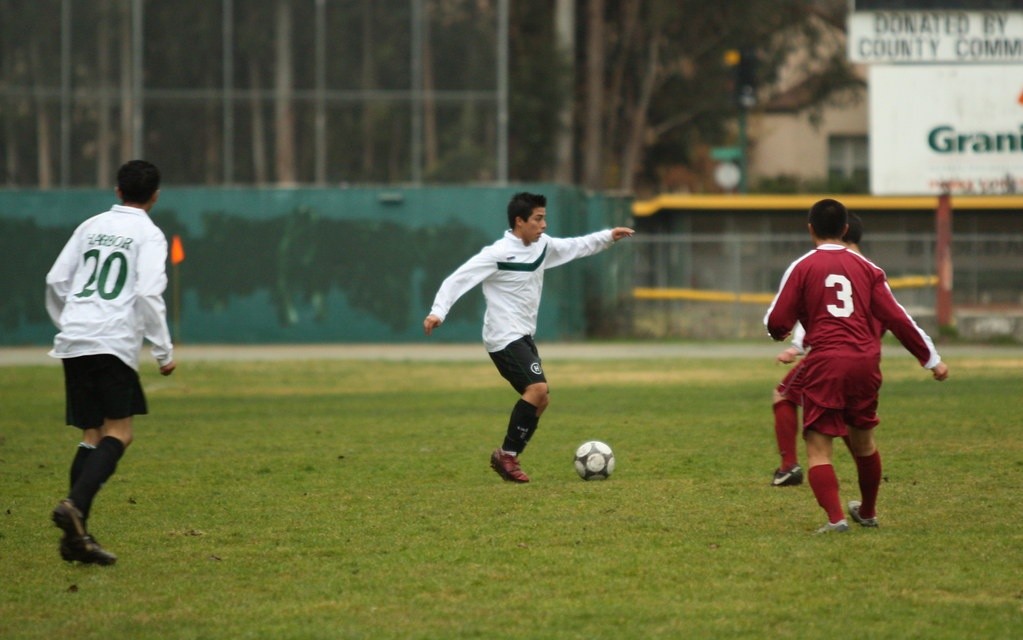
[{"x1": 573, "y1": 439, "x2": 617, "y2": 483}]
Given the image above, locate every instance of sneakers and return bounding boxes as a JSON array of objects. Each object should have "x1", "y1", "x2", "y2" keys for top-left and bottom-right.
[
  {"x1": 489, "y1": 447, "x2": 530, "y2": 484},
  {"x1": 57, "y1": 533, "x2": 118, "y2": 567},
  {"x1": 813, "y1": 519, "x2": 849, "y2": 534},
  {"x1": 847, "y1": 500, "x2": 878, "y2": 527},
  {"x1": 770, "y1": 463, "x2": 803, "y2": 487},
  {"x1": 49, "y1": 497, "x2": 94, "y2": 556}
]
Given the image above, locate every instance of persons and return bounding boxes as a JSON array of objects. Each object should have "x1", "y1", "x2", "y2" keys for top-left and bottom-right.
[
  {"x1": 44, "y1": 160, "x2": 176, "y2": 566},
  {"x1": 769, "y1": 215, "x2": 862, "y2": 487},
  {"x1": 763, "y1": 199, "x2": 948, "y2": 533},
  {"x1": 423, "y1": 192, "x2": 635, "y2": 483}
]
